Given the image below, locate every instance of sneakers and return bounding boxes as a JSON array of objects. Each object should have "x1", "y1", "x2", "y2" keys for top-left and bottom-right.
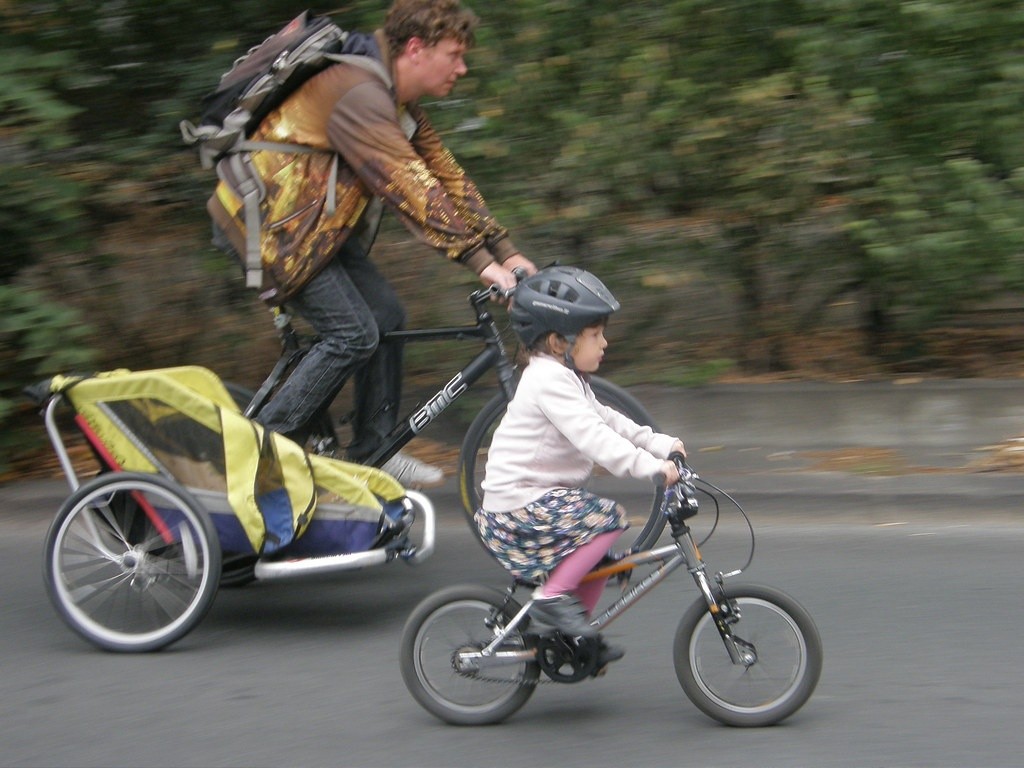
[
  {"x1": 530, "y1": 593, "x2": 597, "y2": 637},
  {"x1": 377, "y1": 452, "x2": 444, "y2": 484},
  {"x1": 585, "y1": 647, "x2": 624, "y2": 676}
]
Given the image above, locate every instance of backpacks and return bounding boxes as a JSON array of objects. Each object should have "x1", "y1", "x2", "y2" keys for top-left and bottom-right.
[{"x1": 179, "y1": 9, "x2": 401, "y2": 170}]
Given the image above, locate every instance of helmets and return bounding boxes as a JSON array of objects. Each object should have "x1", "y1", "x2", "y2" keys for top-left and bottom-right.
[{"x1": 507, "y1": 267, "x2": 620, "y2": 347}]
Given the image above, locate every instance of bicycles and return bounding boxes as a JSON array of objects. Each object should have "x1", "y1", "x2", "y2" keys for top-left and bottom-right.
[
  {"x1": 396, "y1": 448, "x2": 825, "y2": 727},
  {"x1": 24, "y1": 261, "x2": 673, "y2": 655}
]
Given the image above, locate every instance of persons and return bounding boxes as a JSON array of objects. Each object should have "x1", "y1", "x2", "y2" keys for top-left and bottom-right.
[
  {"x1": 204, "y1": 1, "x2": 537, "y2": 487},
  {"x1": 470, "y1": 263, "x2": 687, "y2": 663}
]
